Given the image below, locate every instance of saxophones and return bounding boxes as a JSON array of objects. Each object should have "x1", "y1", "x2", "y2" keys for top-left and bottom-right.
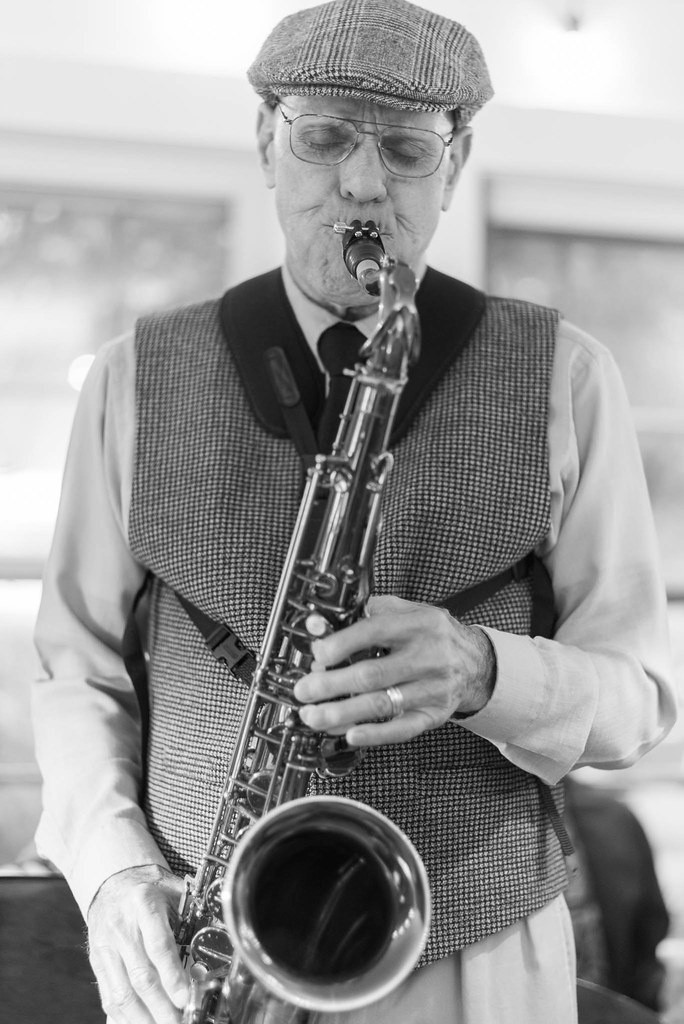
[{"x1": 166, "y1": 220, "x2": 438, "y2": 1024}]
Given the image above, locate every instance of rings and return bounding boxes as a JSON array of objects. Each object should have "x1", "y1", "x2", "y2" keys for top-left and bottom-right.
[{"x1": 386, "y1": 687, "x2": 404, "y2": 723}]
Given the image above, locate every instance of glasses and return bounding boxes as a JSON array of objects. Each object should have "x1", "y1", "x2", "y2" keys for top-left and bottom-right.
[{"x1": 274, "y1": 98, "x2": 454, "y2": 179}]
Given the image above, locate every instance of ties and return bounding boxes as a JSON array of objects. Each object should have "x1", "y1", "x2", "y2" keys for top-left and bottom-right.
[{"x1": 319, "y1": 323, "x2": 371, "y2": 459}]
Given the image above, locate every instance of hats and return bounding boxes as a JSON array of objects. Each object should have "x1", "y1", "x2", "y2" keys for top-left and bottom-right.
[{"x1": 245, "y1": 0, "x2": 494, "y2": 124}]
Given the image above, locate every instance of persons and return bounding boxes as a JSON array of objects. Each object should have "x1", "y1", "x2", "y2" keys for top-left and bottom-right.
[{"x1": 32, "y1": 1, "x2": 672, "y2": 1024}]
